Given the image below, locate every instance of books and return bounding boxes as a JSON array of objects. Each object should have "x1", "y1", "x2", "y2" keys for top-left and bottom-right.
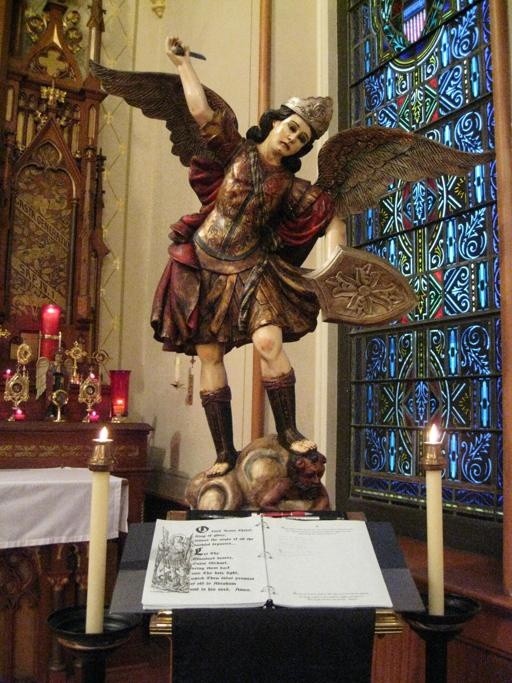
[{"x1": 108, "y1": 514, "x2": 427, "y2": 617}]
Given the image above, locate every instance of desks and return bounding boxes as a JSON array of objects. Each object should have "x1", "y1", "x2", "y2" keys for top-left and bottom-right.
[
  {"x1": 1, "y1": 413, "x2": 154, "y2": 682},
  {"x1": 0, "y1": 462, "x2": 135, "y2": 682}
]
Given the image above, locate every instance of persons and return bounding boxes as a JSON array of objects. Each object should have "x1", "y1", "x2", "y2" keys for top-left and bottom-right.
[
  {"x1": 258, "y1": 451, "x2": 329, "y2": 512},
  {"x1": 85, "y1": 35, "x2": 496, "y2": 478}
]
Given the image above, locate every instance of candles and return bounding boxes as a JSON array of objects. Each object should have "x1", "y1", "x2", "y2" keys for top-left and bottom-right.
[
  {"x1": 113, "y1": 397, "x2": 124, "y2": 416},
  {"x1": 41, "y1": 308, "x2": 58, "y2": 355},
  {"x1": 415, "y1": 420, "x2": 449, "y2": 616},
  {"x1": 81, "y1": 421, "x2": 120, "y2": 636}
]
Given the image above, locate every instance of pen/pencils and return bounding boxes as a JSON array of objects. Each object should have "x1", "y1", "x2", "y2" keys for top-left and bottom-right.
[{"x1": 247, "y1": 510, "x2": 316, "y2": 517}]
